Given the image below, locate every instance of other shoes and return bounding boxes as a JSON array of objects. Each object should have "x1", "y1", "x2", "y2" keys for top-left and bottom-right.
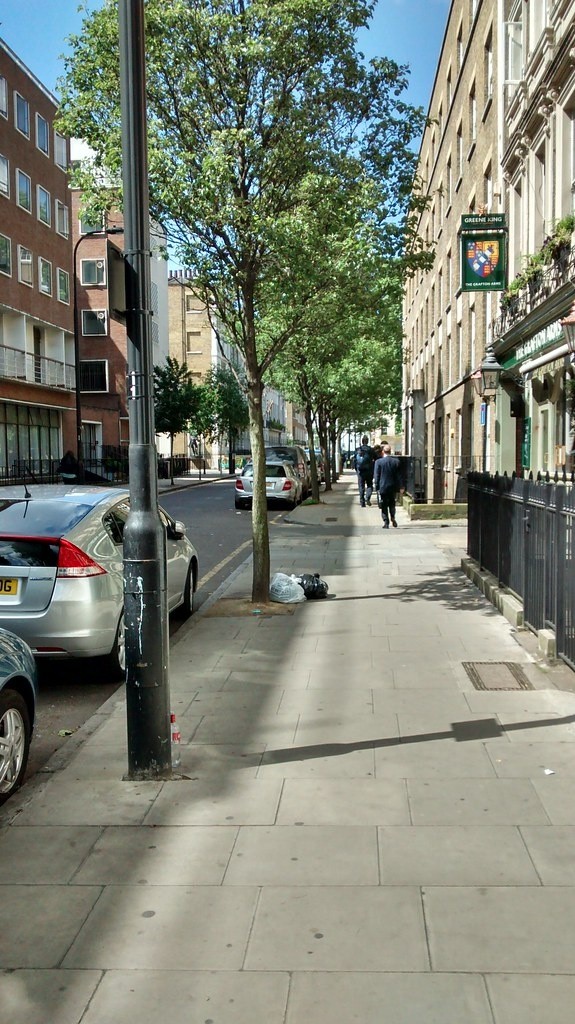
[
  {"x1": 382, "y1": 524, "x2": 388, "y2": 528},
  {"x1": 391, "y1": 518, "x2": 397, "y2": 527}
]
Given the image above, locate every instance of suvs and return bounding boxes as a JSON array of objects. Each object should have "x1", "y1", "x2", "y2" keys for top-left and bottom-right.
[{"x1": 263, "y1": 444, "x2": 311, "y2": 499}]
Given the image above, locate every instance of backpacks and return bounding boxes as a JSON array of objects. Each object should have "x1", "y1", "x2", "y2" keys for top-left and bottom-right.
[{"x1": 356, "y1": 446, "x2": 372, "y2": 476}]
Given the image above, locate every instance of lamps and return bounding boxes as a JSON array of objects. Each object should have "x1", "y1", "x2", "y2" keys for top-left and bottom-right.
[
  {"x1": 559, "y1": 299, "x2": 575, "y2": 366},
  {"x1": 482, "y1": 346, "x2": 505, "y2": 391},
  {"x1": 470, "y1": 369, "x2": 488, "y2": 398}
]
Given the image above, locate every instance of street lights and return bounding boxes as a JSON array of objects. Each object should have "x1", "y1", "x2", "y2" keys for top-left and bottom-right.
[{"x1": 72, "y1": 225, "x2": 124, "y2": 465}]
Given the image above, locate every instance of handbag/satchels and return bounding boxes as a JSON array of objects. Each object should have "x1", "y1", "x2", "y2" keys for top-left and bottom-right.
[{"x1": 376, "y1": 491, "x2": 384, "y2": 509}]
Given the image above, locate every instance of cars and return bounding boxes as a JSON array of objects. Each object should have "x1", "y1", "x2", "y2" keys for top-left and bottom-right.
[
  {"x1": 305, "y1": 449, "x2": 332, "y2": 485},
  {"x1": 234, "y1": 458, "x2": 302, "y2": 509},
  {"x1": 0, "y1": 460, "x2": 199, "y2": 681}
]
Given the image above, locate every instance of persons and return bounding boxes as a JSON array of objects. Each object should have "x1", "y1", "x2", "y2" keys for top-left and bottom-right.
[
  {"x1": 374, "y1": 445, "x2": 382, "y2": 459},
  {"x1": 352, "y1": 435, "x2": 375, "y2": 507},
  {"x1": 61, "y1": 450, "x2": 83, "y2": 485},
  {"x1": 374, "y1": 445, "x2": 404, "y2": 529},
  {"x1": 381, "y1": 441, "x2": 389, "y2": 444}
]
